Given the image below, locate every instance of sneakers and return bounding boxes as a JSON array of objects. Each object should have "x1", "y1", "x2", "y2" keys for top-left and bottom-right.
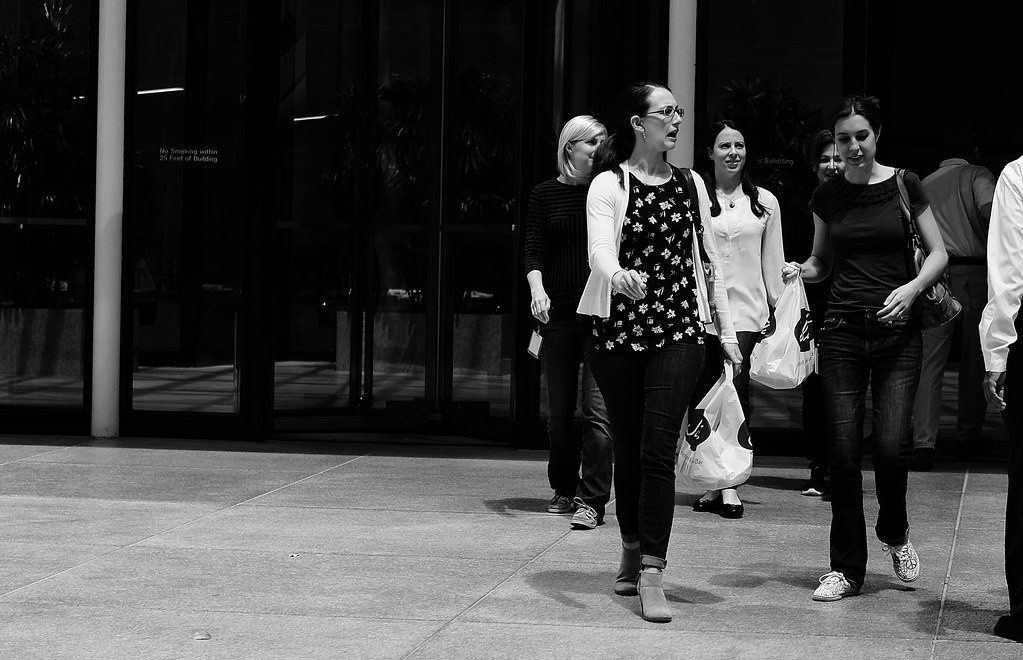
[
  {"x1": 812, "y1": 571, "x2": 860, "y2": 601},
  {"x1": 882, "y1": 535, "x2": 919, "y2": 583},
  {"x1": 547, "y1": 494, "x2": 572, "y2": 513},
  {"x1": 571, "y1": 497, "x2": 603, "y2": 529}
]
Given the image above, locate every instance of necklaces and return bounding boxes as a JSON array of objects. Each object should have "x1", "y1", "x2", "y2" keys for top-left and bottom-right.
[{"x1": 718, "y1": 185, "x2": 739, "y2": 208}]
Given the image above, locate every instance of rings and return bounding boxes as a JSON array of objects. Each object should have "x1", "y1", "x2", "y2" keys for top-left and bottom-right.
[{"x1": 898, "y1": 312, "x2": 903, "y2": 319}]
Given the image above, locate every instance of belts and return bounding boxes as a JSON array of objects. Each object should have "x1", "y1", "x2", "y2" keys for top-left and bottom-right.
[{"x1": 948, "y1": 257, "x2": 986, "y2": 266}]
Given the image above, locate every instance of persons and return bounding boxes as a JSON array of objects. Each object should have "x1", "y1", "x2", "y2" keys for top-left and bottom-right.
[
  {"x1": 978, "y1": 155, "x2": 1023, "y2": 643},
  {"x1": 788, "y1": 130, "x2": 845, "y2": 496},
  {"x1": 522, "y1": 113, "x2": 614, "y2": 529},
  {"x1": 575, "y1": 83, "x2": 744, "y2": 624},
  {"x1": 691, "y1": 119, "x2": 786, "y2": 520},
  {"x1": 911, "y1": 128, "x2": 996, "y2": 472},
  {"x1": 780, "y1": 92, "x2": 948, "y2": 601}
]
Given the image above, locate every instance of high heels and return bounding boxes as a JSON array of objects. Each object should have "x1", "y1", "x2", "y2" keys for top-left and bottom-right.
[
  {"x1": 614, "y1": 541, "x2": 640, "y2": 594},
  {"x1": 637, "y1": 567, "x2": 672, "y2": 621}
]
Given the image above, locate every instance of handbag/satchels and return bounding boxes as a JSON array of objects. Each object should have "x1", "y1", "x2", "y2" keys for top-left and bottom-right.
[
  {"x1": 893, "y1": 167, "x2": 962, "y2": 332},
  {"x1": 675, "y1": 360, "x2": 753, "y2": 495},
  {"x1": 749, "y1": 261, "x2": 819, "y2": 390}
]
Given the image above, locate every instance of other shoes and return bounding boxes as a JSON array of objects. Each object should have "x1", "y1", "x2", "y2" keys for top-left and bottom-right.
[
  {"x1": 718, "y1": 492, "x2": 744, "y2": 518},
  {"x1": 948, "y1": 435, "x2": 994, "y2": 463},
  {"x1": 801, "y1": 466, "x2": 827, "y2": 495},
  {"x1": 695, "y1": 490, "x2": 722, "y2": 511},
  {"x1": 994, "y1": 615, "x2": 1023, "y2": 643},
  {"x1": 909, "y1": 449, "x2": 935, "y2": 472}
]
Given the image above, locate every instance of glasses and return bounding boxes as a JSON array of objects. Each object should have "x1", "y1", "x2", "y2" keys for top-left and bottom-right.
[{"x1": 639, "y1": 105, "x2": 685, "y2": 118}]
